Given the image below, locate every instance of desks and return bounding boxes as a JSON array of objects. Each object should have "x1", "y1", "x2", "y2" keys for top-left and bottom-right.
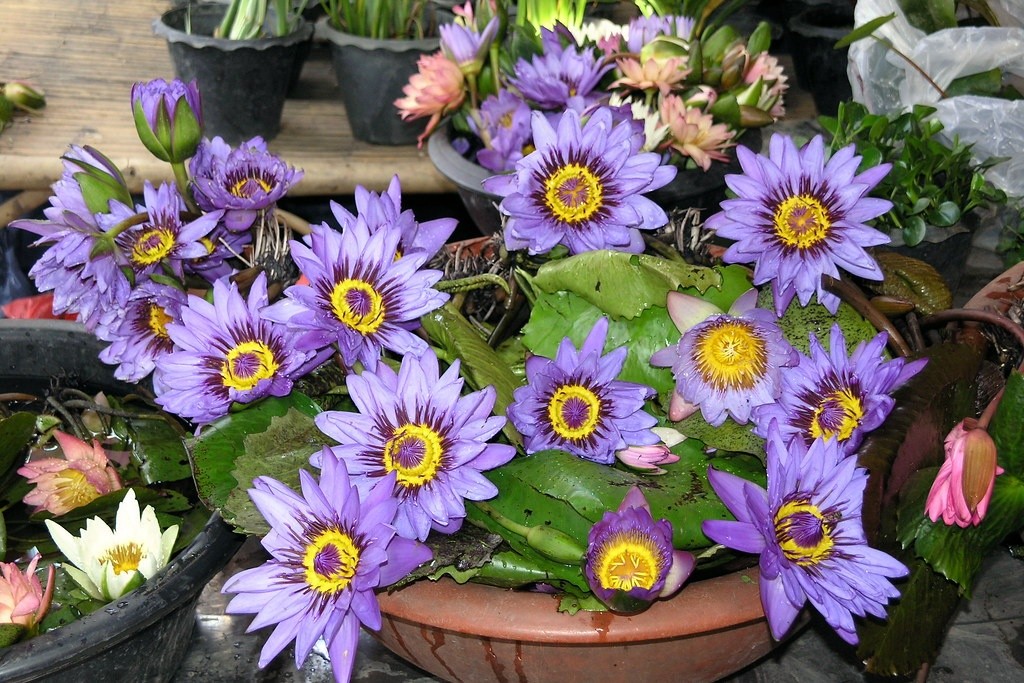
[{"x1": 0, "y1": 0, "x2": 464, "y2": 238}]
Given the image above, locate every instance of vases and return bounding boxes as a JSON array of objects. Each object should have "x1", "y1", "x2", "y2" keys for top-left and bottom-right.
[
  {"x1": 886, "y1": 260, "x2": 1023, "y2": 499},
  {"x1": 2, "y1": 318, "x2": 247, "y2": 683},
  {"x1": 428, "y1": 123, "x2": 762, "y2": 236},
  {"x1": 361, "y1": 235, "x2": 911, "y2": 683}
]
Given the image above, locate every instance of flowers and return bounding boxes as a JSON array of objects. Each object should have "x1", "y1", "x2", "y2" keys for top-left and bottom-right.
[{"x1": 0, "y1": 0, "x2": 1024, "y2": 683}]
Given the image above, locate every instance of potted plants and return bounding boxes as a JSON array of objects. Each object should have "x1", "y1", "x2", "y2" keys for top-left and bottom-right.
[
  {"x1": 151, "y1": 1, "x2": 315, "y2": 143},
  {"x1": 307, "y1": 0, "x2": 447, "y2": 145},
  {"x1": 801, "y1": 100, "x2": 1013, "y2": 297}
]
